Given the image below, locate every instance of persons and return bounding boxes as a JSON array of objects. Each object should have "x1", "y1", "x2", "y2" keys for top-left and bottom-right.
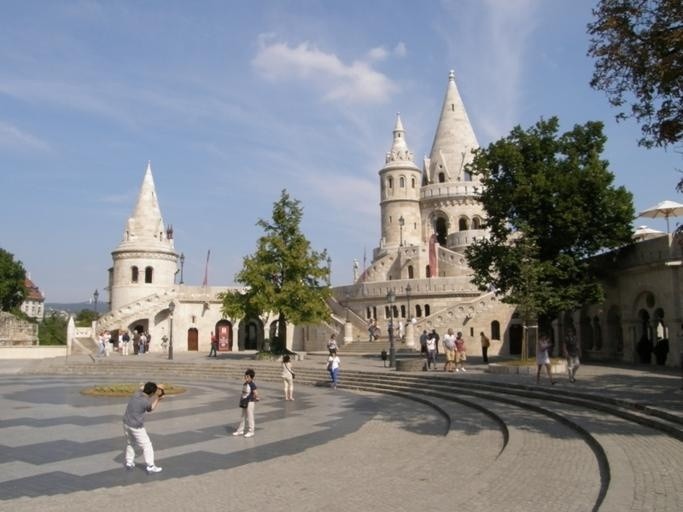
[
  {"x1": 327, "y1": 349, "x2": 341, "y2": 390},
  {"x1": 282, "y1": 356, "x2": 295, "y2": 400},
  {"x1": 161, "y1": 335, "x2": 168, "y2": 352},
  {"x1": 232, "y1": 369, "x2": 258, "y2": 438},
  {"x1": 536, "y1": 332, "x2": 558, "y2": 385},
  {"x1": 368, "y1": 315, "x2": 467, "y2": 372},
  {"x1": 122, "y1": 382, "x2": 163, "y2": 472},
  {"x1": 480, "y1": 331, "x2": 490, "y2": 362},
  {"x1": 97, "y1": 329, "x2": 151, "y2": 356},
  {"x1": 327, "y1": 334, "x2": 339, "y2": 356},
  {"x1": 208, "y1": 332, "x2": 217, "y2": 357},
  {"x1": 563, "y1": 328, "x2": 581, "y2": 383}
]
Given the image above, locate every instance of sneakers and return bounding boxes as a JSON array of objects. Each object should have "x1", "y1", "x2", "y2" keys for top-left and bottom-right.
[
  {"x1": 146, "y1": 465, "x2": 162, "y2": 473},
  {"x1": 126, "y1": 462, "x2": 135, "y2": 468},
  {"x1": 232, "y1": 430, "x2": 255, "y2": 437}
]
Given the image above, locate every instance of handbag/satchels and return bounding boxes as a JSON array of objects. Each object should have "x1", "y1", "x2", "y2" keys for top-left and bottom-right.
[{"x1": 239, "y1": 397, "x2": 247, "y2": 408}]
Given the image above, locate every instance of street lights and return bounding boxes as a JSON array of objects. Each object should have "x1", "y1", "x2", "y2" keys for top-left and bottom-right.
[
  {"x1": 386, "y1": 289, "x2": 397, "y2": 368},
  {"x1": 166, "y1": 301, "x2": 177, "y2": 361},
  {"x1": 398, "y1": 215, "x2": 404, "y2": 246},
  {"x1": 404, "y1": 280, "x2": 412, "y2": 322},
  {"x1": 325, "y1": 256, "x2": 332, "y2": 288},
  {"x1": 178, "y1": 250, "x2": 185, "y2": 285},
  {"x1": 92, "y1": 289, "x2": 100, "y2": 312}
]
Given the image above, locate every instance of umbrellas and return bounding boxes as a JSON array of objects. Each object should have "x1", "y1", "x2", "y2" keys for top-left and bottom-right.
[
  {"x1": 630, "y1": 226, "x2": 667, "y2": 242},
  {"x1": 639, "y1": 200, "x2": 683, "y2": 233}
]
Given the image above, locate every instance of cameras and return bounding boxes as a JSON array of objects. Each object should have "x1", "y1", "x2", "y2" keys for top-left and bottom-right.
[{"x1": 158, "y1": 389, "x2": 164, "y2": 397}]
